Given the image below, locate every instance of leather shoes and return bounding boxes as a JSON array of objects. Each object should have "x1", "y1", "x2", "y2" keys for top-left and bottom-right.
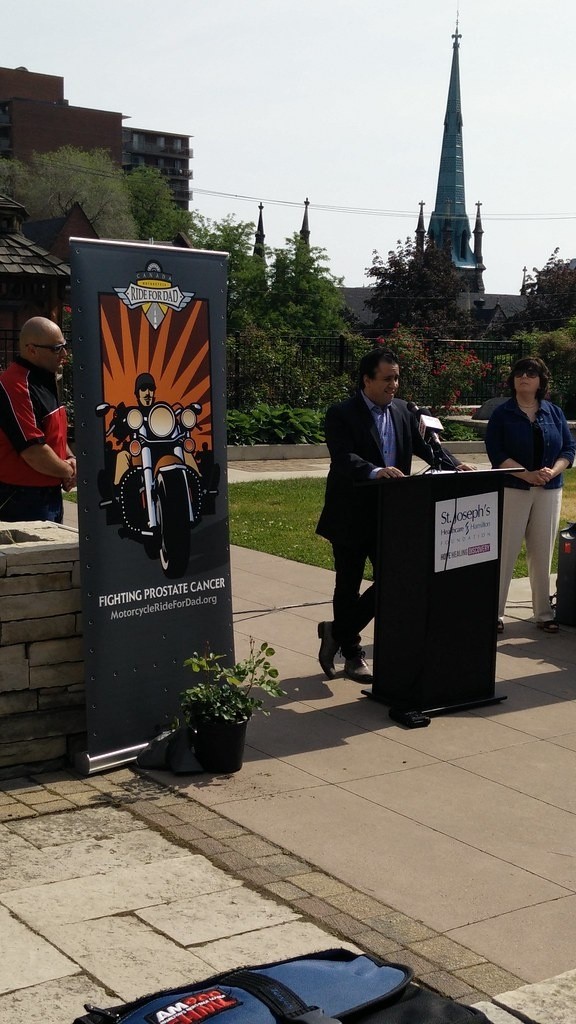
[
  {"x1": 342, "y1": 655, "x2": 374, "y2": 684},
  {"x1": 318, "y1": 621, "x2": 339, "y2": 679}
]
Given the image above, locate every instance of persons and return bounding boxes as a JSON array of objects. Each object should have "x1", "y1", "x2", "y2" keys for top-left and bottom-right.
[
  {"x1": 0, "y1": 317, "x2": 77, "y2": 523},
  {"x1": 315, "y1": 347, "x2": 477, "y2": 683},
  {"x1": 485, "y1": 358, "x2": 576, "y2": 633}
]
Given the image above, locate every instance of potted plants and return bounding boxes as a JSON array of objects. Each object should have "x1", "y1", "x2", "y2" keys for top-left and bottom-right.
[{"x1": 169, "y1": 634, "x2": 283, "y2": 774}]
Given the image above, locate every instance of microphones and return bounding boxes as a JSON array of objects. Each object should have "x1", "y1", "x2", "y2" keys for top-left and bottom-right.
[
  {"x1": 415, "y1": 408, "x2": 444, "y2": 449},
  {"x1": 406, "y1": 402, "x2": 420, "y2": 413}
]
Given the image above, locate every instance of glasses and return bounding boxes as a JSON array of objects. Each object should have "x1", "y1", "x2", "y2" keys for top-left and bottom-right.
[
  {"x1": 25, "y1": 338, "x2": 69, "y2": 353},
  {"x1": 511, "y1": 367, "x2": 540, "y2": 379}
]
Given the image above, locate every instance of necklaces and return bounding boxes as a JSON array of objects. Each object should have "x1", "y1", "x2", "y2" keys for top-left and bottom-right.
[{"x1": 517, "y1": 397, "x2": 537, "y2": 408}]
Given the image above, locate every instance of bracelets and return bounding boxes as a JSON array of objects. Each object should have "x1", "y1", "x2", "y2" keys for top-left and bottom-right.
[{"x1": 66, "y1": 456, "x2": 77, "y2": 459}]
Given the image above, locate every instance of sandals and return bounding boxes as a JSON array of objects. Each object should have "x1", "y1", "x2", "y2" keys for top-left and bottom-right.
[
  {"x1": 536, "y1": 618, "x2": 559, "y2": 632},
  {"x1": 496, "y1": 618, "x2": 506, "y2": 633}
]
